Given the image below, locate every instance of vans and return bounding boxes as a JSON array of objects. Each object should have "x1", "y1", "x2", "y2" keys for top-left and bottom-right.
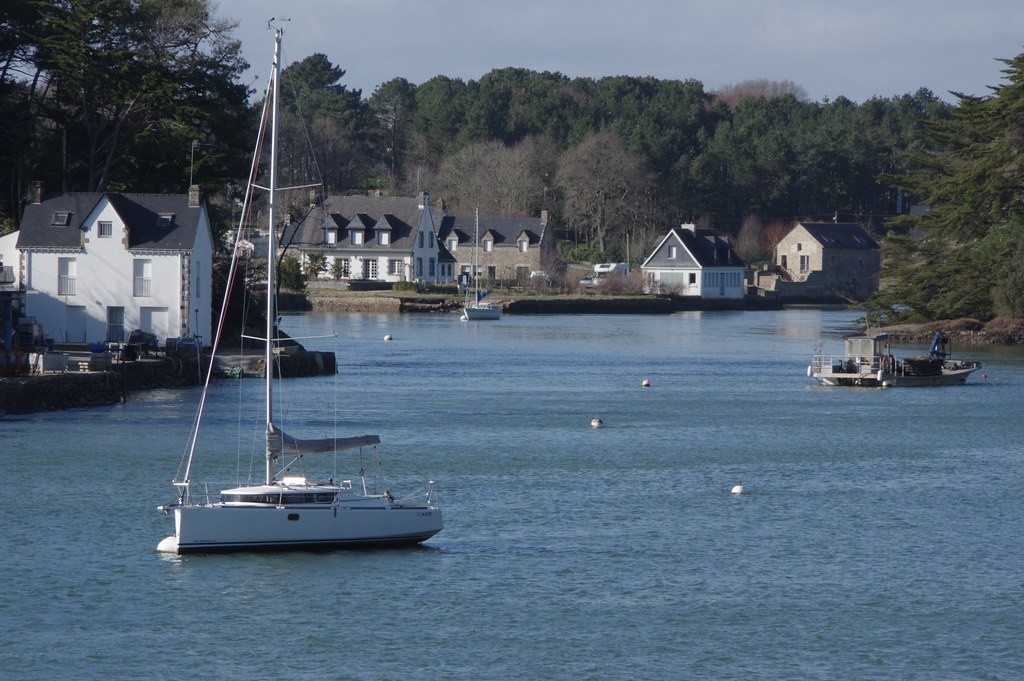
[{"x1": 592, "y1": 261, "x2": 631, "y2": 291}]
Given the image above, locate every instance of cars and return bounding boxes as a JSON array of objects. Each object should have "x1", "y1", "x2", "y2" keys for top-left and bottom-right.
[{"x1": 579, "y1": 275, "x2": 594, "y2": 289}]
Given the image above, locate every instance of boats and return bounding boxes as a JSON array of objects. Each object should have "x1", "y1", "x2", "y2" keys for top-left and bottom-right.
[{"x1": 806, "y1": 312, "x2": 984, "y2": 388}]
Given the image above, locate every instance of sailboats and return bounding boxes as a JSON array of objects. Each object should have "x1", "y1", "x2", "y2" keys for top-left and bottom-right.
[
  {"x1": 156, "y1": 16, "x2": 447, "y2": 555},
  {"x1": 463, "y1": 199, "x2": 503, "y2": 321}
]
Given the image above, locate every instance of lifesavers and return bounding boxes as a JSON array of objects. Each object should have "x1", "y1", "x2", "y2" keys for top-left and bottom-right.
[{"x1": 881, "y1": 357, "x2": 889, "y2": 370}]
